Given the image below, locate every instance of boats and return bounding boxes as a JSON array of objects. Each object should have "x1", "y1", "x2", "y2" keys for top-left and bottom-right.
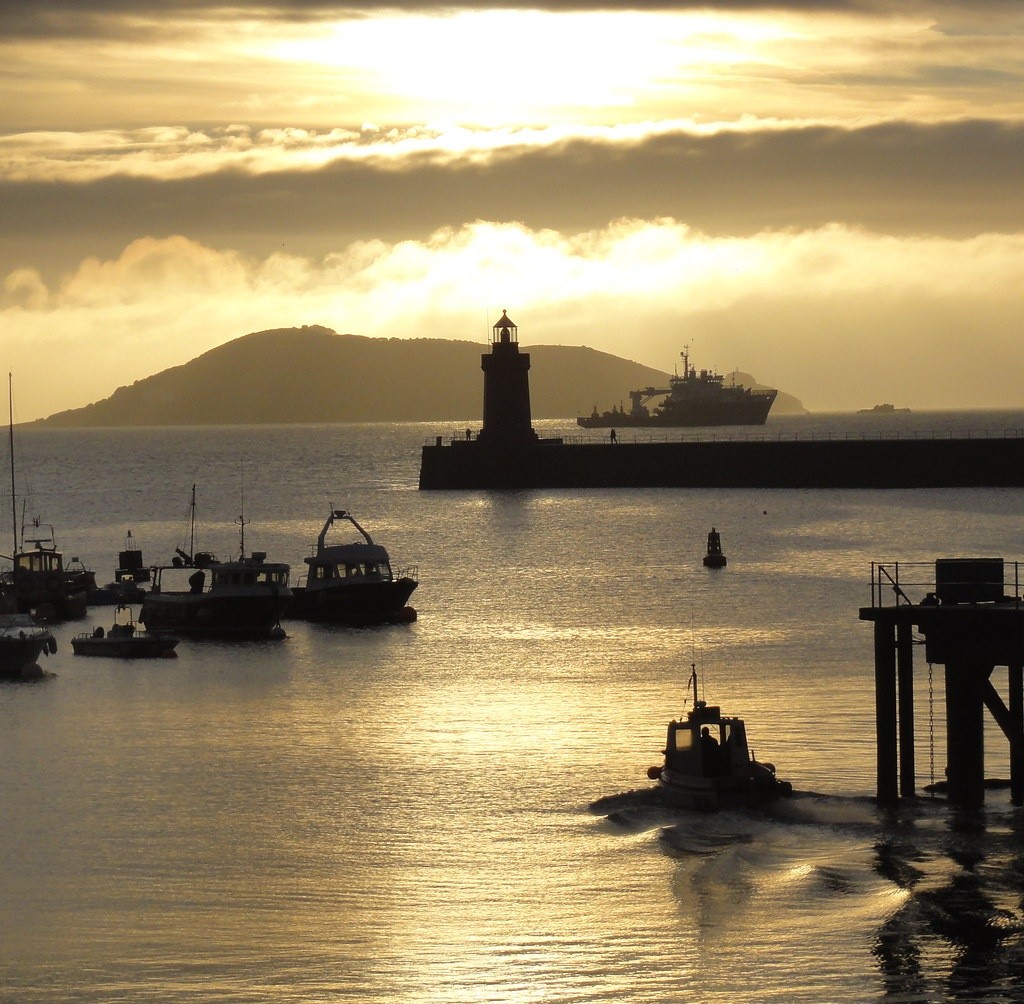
[
  {"x1": 1, "y1": 370, "x2": 156, "y2": 621},
  {"x1": 71, "y1": 598, "x2": 181, "y2": 658},
  {"x1": 0, "y1": 623, "x2": 58, "y2": 673},
  {"x1": 647, "y1": 611, "x2": 776, "y2": 812},
  {"x1": 139, "y1": 466, "x2": 293, "y2": 625},
  {"x1": 293, "y1": 497, "x2": 420, "y2": 615},
  {"x1": 576, "y1": 337, "x2": 779, "y2": 429}
]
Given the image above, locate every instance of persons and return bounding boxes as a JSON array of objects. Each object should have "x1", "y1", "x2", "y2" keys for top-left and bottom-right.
[
  {"x1": 611, "y1": 429, "x2": 617, "y2": 444},
  {"x1": 466, "y1": 429, "x2": 471, "y2": 441},
  {"x1": 702, "y1": 727, "x2": 718, "y2": 752}
]
